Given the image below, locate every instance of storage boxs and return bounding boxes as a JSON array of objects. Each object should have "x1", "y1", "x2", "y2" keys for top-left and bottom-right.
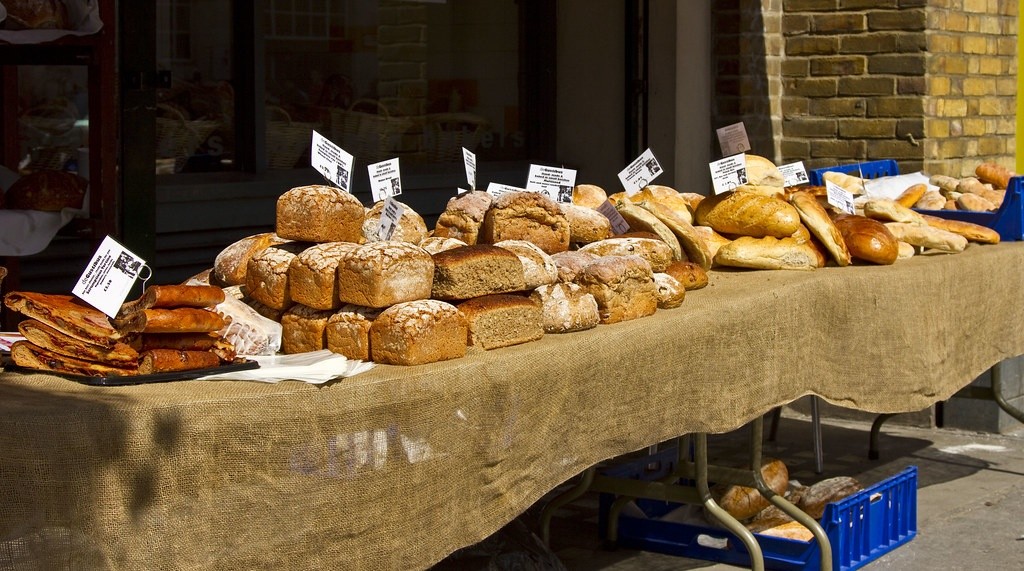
[
  {"x1": 594, "y1": 446, "x2": 918, "y2": 571},
  {"x1": 809, "y1": 161, "x2": 1024, "y2": 240}
]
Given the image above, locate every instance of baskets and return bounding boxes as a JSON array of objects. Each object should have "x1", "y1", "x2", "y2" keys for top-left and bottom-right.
[
  {"x1": 810, "y1": 158, "x2": 1024, "y2": 241},
  {"x1": 600, "y1": 444, "x2": 917, "y2": 571}
]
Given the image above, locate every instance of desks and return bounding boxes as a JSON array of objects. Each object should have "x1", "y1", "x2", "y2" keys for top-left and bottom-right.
[{"x1": 1, "y1": 238, "x2": 1024, "y2": 571}]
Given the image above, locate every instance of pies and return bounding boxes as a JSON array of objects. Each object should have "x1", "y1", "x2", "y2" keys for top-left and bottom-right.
[{"x1": 3, "y1": 282, "x2": 246, "y2": 380}]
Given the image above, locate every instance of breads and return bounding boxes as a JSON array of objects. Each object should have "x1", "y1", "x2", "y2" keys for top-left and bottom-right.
[
  {"x1": 180, "y1": 184, "x2": 713, "y2": 367},
  {"x1": 606, "y1": 154, "x2": 1019, "y2": 273},
  {"x1": 703, "y1": 457, "x2": 867, "y2": 544}
]
